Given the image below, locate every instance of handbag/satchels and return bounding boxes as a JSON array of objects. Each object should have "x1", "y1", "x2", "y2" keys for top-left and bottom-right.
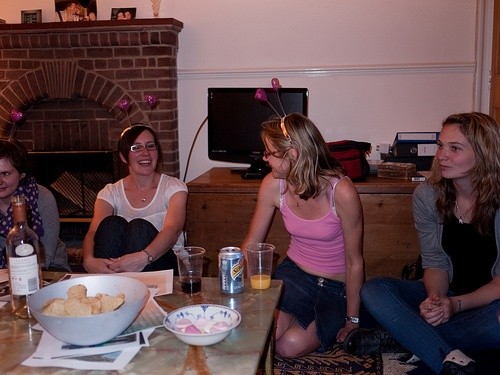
[{"x1": 325, "y1": 138, "x2": 372, "y2": 181}]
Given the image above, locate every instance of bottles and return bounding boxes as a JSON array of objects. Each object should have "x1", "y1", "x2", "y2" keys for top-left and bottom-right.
[{"x1": 5, "y1": 196, "x2": 44, "y2": 321}]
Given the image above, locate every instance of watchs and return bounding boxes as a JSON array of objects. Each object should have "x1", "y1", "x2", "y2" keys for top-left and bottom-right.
[
  {"x1": 343, "y1": 314, "x2": 362, "y2": 325},
  {"x1": 142, "y1": 249, "x2": 153, "y2": 266}
]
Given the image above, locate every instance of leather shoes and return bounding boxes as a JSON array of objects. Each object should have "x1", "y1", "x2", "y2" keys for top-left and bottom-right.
[
  {"x1": 433, "y1": 358, "x2": 484, "y2": 375},
  {"x1": 344, "y1": 326, "x2": 414, "y2": 356}
]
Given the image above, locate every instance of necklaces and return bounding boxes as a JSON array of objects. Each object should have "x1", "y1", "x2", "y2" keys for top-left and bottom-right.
[
  {"x1": 285, "y1": 182, "x2": 306, "y2": 208},
  {"x1": 130, "y1": 172, "x2": 157, "y2": 203},
  {"x1": 454, "y1": 196, "x2": 479, "y2": 226}
]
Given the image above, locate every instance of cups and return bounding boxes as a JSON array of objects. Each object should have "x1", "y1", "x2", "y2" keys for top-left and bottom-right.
[
  {"x1": 245, "y1": 242, "x2": 275, "y2": 291},
  {"x1": 173, "y1": 246, "x2": 206, "y2": 296}
]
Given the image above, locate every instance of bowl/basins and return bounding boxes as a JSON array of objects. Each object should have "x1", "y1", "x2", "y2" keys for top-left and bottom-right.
[
  {"x1": 163, "y1": 303, "x2": 242, "y2": 347},
  {"x1": 27, "y1": 276, "x2": 149, "y2": 346}
]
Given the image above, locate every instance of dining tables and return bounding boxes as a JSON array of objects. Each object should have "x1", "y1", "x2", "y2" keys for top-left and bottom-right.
[{"x1": 0, "y1": 272, "x2": 283, "y2": 374}]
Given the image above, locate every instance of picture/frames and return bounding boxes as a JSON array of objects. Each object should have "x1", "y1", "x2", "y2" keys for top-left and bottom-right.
[
  {"x1": 21, "y1": 9, "x2": 42, "y2": 24},
  {"x1": 54, "y1": 0, "x2": 97, "y2": 22},
  {"x1": 111, "y1": 7, "x2": 137, "y2": 20}
]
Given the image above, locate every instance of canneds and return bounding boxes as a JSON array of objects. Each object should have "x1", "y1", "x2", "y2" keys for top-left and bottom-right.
[{"x1": 218, "y1": 246, "x2": 244, "y2": 294}]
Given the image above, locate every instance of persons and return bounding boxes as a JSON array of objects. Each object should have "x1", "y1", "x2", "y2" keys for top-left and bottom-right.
[
  {"x1": 238, "y1": 112, "x2": 364, "y2": 360},
  {"x1": 116, "y1": 11, "x2": 133, "y2": 20},
  {"x1": 359, "y1": 112, "x2": 500, "y2": 375},
  {"x1": 0, "y1": 137, "x2": 72, "y2": 274},
  {"x1": 82, "y1": 122, "x2": 190, "y2": 278}
]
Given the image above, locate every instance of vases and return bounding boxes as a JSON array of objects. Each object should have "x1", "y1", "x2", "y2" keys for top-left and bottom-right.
[{"x1": 151, "y1": 0, "x2": 161, "y2": 18}]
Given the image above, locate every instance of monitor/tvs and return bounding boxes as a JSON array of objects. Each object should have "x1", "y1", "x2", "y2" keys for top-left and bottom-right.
[{"x1": 206, "y1": 88, "x2": 309, "y2": 165}]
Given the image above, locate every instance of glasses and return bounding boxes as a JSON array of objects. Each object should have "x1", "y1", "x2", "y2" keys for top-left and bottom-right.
[
  {"x1": 263, "y1": 149, "x2": 286, "y2": 159},
  {"x1": 129, "y1": 143, "x2": 158, "y2": 150}
]
